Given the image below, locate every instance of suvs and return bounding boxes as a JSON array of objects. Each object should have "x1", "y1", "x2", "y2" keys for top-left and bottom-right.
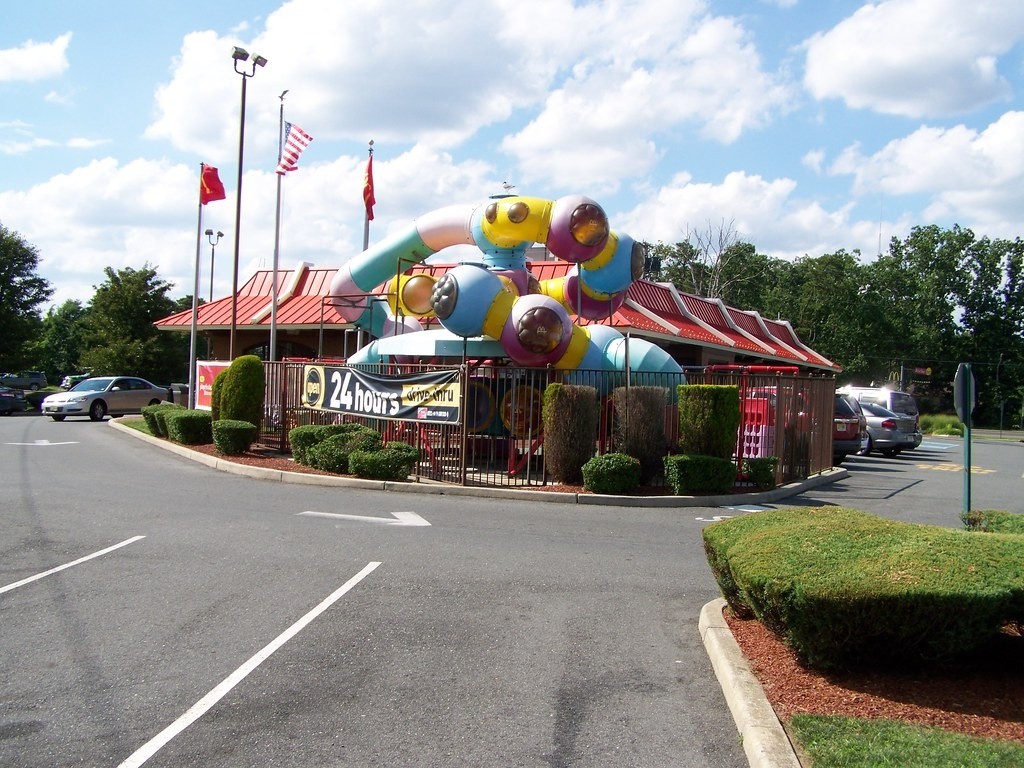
[{"x1": 739, "y1": 385, "x2": 861, "y2": 468}]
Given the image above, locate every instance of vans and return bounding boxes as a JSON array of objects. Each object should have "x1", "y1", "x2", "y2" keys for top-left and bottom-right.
[{"x1": 835, "y1": 386, "x2": 919, "y2": 429}]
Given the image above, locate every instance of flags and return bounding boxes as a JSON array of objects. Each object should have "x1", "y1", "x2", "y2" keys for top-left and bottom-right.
[
  {"x1": 362, "y1": 156, "x2": 376, "y2": 221},
  {"x1": 200, "y1": 163, "x2": 226, "y2": 205},
  {"x1": 275, "y1": 121, "x2": 313, "y2": 175}
]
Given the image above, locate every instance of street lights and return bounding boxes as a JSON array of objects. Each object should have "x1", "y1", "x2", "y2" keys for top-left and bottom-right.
[
  {"x1": 204, "y1": 229, "x2": 224, "y2": 361},
  {"x1": 229, "y1": 45, "x2": 269, "y2": 361}
]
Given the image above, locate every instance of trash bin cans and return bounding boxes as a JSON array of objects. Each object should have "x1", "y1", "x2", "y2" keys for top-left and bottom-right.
[{"x1": 171, "y1": 383, "x2": 189, "y2": 408}]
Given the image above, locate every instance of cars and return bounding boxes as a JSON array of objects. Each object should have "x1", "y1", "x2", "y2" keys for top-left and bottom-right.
[
  {"x1": 42, "y1": 376, "x2": 168, "y2": 422},
  {"x1": 60, "y1": 370, "x2": 96, "y2": 391},
  {"x1": 856, "y1": 401, "x2": 923, "y2": 458},
  {"x1": 0, "y1": 371, "x2": 48, "y2": 391}
]
